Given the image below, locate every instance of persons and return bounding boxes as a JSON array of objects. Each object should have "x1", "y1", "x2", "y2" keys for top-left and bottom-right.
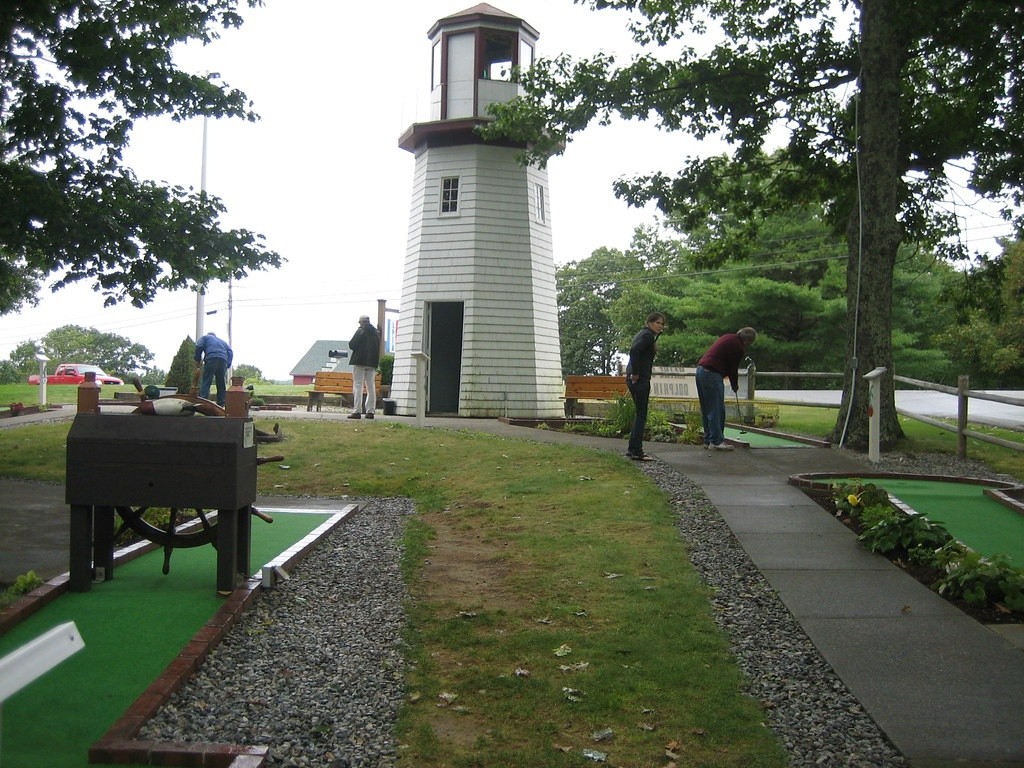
[
  {"x1": 347, "y1": 314, "x2": 381, "y2": 419},
  {"x1": 194, "y1": 331, "x2": 233, "y2": 410},
  {"x1": 694, "y1": 326, "x2": 759, "y2": 452},
  {"x1": 625, "y1": 313, "x2": 665, "y2": 462}
]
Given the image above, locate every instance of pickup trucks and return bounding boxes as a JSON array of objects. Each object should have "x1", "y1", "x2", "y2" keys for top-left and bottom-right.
[{"x1": 28, "y1": 364, "x2": 124, "y2": 386}]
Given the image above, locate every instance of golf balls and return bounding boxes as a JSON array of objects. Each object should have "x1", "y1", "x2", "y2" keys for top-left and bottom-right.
[{"x1": 737, "y1": 437, "x2": 740, "y2": 440}]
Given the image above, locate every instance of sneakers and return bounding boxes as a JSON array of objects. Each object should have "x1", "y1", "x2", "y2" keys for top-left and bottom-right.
[
  {"x1": 703, "y1": 441, "x2": 710, "y2": 449},
  {"x1": 708, "y1": 442, "x2": 734, "y2": 451}
]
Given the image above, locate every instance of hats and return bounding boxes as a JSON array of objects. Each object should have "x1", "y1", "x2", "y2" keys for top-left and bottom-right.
[{"x1": 357, "y1": 315, "x2": 369, "y2": 323}]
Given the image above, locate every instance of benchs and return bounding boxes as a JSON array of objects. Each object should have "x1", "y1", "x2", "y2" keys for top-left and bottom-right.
[
  {"x1": 304, "y1": 372, "x2": 383, "y2": 414},
  {"x1": 559, "y1": 376, "x2": 632, "y2": 418}
]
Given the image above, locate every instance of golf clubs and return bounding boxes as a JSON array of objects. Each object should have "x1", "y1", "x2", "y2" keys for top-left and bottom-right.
[
  {"x1": 735, "y1": 390, "x2": 747, "y2": 435},
  {"x1": 620, "y1": 330, "x2": 667, "y2": 405}
]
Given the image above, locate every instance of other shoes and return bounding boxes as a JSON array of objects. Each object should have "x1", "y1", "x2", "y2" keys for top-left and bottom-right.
[
  {"x1": 365, "y1": 413, "x2": 374, "y2": 419},
  {"x1": 632, "y1": 453, "x2": 653, "y2": 461},
  {"x1": 626, "y1": 450, "x2": 632, "y2": 457},
  {"x1": 348, "y1": 413, "x2": 361, "y2": 419}
]
